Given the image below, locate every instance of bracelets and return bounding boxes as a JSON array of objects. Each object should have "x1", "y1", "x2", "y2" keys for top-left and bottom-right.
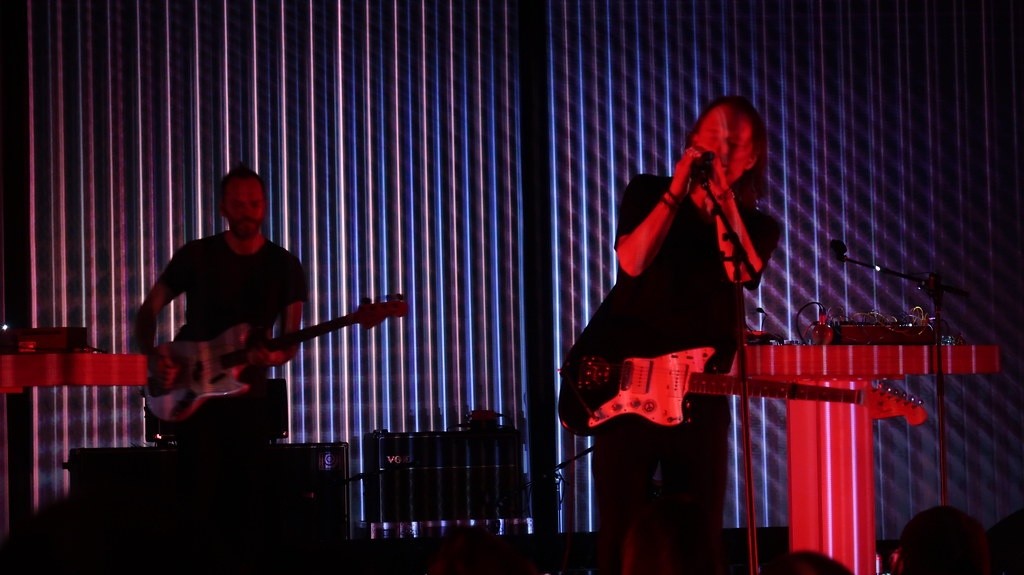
[
  {"x1": 716, "y1": 189, "x2": 735, "y2": 203},
  {"x1": 662, "y1": 190, "x2": 681, "y2": 215}
]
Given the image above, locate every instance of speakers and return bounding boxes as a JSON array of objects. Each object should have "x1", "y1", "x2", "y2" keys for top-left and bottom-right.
[
  {"x1": 68, "y1": 440, "x2": 352, "y2": 575},
  {"x1": 364, "y1": 432, "x2": 526, "y2": 523}
]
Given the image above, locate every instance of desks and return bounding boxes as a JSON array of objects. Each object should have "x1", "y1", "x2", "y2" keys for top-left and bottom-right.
[{"x1": 732, "y1": 344, "x2": 1001, "y2": 575}]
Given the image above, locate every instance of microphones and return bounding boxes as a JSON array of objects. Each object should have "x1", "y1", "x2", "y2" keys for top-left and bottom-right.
[{"x1": 691, "y1": 151, "x2": 715, "y2": 177}]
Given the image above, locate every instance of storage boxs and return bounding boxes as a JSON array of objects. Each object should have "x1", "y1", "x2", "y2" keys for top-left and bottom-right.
[{"x1": 0, "y1": 353, "x2": 147, "y2": 544}]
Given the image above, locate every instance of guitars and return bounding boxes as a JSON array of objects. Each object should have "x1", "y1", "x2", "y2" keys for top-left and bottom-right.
[
  {"x1": 552, "y1": 330, "x2": 930, "y2": 439},
  {"x1": 144, "y1": 288, "x2": 410, "y2": 423}
]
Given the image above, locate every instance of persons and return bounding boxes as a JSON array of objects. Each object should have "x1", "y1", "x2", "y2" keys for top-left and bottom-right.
[
  {"x1": 133, "y1": 167, "x2": 308, "y2": 498},
  {"x1": 592, "y1": 95, "x2": 783, "y2": 575},
  {"x1": 900, "y1": 506, "x2": 989, "y2": 574}
]
